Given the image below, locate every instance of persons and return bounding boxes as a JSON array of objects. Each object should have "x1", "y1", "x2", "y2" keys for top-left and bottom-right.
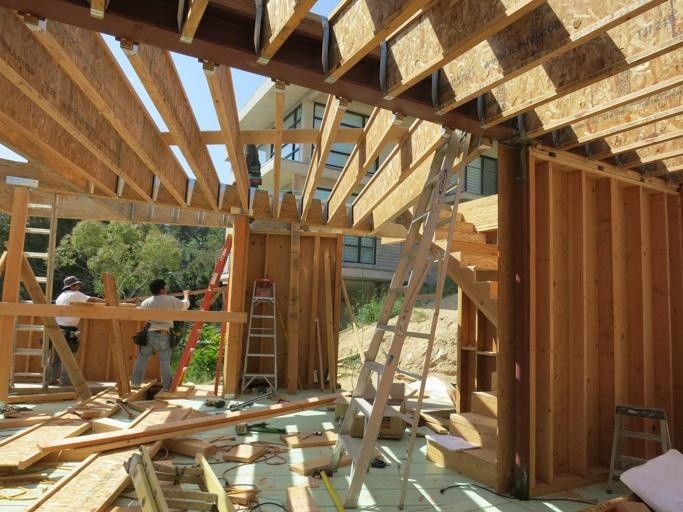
[
  {"x1": 230, "y1": 144, "x2": 261, "y2": 188},
  {"x1": 130, "y1": 278, "x2": 190, "y2": 389},
  {"x1": 49, "y1": 275, "x2": 107, "y2": 386}
]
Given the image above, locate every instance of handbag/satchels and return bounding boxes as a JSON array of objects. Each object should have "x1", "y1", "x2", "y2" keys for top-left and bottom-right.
[{"x1": 131, "y1": 329, "x2": 148, "y2": 345}]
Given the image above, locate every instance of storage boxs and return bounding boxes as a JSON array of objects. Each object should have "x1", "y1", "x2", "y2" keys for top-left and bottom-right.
[
  {"x1": 335, "y1": 402, "x2": 354, "y2": 422},
  {"x1": 355, "y1": 399, "x2": 407, "y2": 440}
]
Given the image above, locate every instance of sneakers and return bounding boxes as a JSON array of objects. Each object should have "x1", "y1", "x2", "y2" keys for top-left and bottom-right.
[
  {"x1": 47, "y1": 380, "x2": 60, "y2": 385},
  {"x1": 61, "y1": 381, "x2": 72, "y2": 386}
]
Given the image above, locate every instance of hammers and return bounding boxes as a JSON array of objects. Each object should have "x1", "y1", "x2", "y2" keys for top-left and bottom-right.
[{"x1": 311, "y1": 464, "x2": 347, "y2": 512}]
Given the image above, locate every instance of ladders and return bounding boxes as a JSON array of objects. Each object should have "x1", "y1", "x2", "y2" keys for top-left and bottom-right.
[
  {"x1": 326, "y1": 127, "x2": 470, "y2": 510},
  {"x1": 9, "y1": 193, "x2": 60, "y2": 389},
  {"x1": 123, "y1": 444, "x2": 237, "y2": 512},
  {"x1": 240, "y1": 278, "x2": 278, "y2": 397},
  {"x1": 170, "y1": 233, "x2": 233, "y2": 394}
]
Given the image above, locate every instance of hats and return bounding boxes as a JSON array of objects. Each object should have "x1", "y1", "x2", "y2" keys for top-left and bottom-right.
[{"x1": 62, "y1": 276, "x2": 81, "y2": 290}]
